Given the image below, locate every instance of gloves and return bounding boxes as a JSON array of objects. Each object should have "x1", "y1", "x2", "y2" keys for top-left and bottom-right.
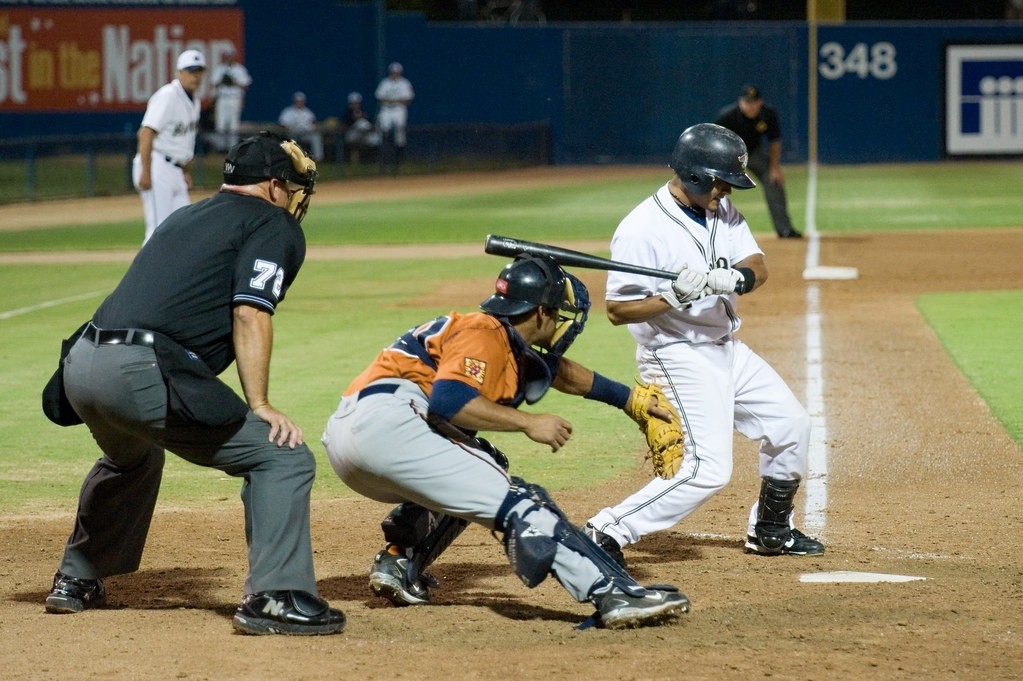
[
  {"x1": 661, "y1": 262, "x2": 709, "y2": 312},
  {"x1": 704, "y1": 266, "x2": 746, "y2": 296}
]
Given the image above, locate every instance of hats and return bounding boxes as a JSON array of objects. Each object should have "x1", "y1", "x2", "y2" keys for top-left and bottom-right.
[
  {"x1": 294, "y1": 92, "x2": 305, "y2": 102},
  {"x1": 388, "y1": 62, "x2": 403, "y2": 73},
  {"x1": 348, "y1": 92, "x2": 363, "y2": 104},
  {"x1": 176, "y1": 50, "x2": 206, "y2": 72},
  {"x1": 738, "y1": 84, "x2": 760, "y2": 102}
]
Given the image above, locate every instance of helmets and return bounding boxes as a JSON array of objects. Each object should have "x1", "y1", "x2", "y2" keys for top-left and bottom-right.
[
  {"x1": 223, "y1": 130, "x2": 292, "y2": 181},
  {"x1": 479, "y1": 253, "x2": 566, "y2": 316},
  {"x1": 666, "y1": 123, "x2": 756, "y2": 194}
]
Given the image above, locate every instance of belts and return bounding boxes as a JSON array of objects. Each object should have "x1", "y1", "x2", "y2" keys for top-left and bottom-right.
[
  {"x1": 82, "y1": 324, "x2": 154, "y2": 348},
  {"x1": 357, "y1": 383, "x2": 400, "y2": 401},
  {"x1": 166, "y1": 155, "x2": 181, "y2": 168}
]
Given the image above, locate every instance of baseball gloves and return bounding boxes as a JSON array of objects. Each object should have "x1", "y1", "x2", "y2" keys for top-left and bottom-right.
[{"x1": 629, "y1": 383, "x2": 686, "y2": 481}]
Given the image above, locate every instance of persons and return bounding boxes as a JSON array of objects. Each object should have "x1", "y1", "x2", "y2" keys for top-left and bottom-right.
[
  {"x1": 42, "y1": 128, "x2": 347, "y2": 636},
  {"x1": 579, "y1": 123, "x2": 826, "y2": 569},
  {"x1": 713, "y1": 84, "x2": 802, "y2": 239},
  {"x1": 319, "y1": 253, "x2": 691, "y2": 630},
  {"x1": 210, "y1": 44, "x2": 415, "y2": 168},
  {"x1": 133, "y1": 50, "x2": 210, "y2": 250}
]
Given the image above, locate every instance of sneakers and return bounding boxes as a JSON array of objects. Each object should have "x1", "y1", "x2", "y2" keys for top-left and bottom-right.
[
  {"x1": 369, "y1": 550, "x2": 430, "y2": 606},
  {"x1": 777, "y1": 228, "x2": 801, "y2": 239},
  {"x1": 45, "y1": 572, "x2": 105, "y2": 614},
  {"x1": 579, "y1": 523, "x2": 627, "y2": 572},
  {"x1": 743, "y1": 528, "x2": 825, "y2": 557},
  {"x1": 587, "y1": 583, "x2": 690, "y2": 631},
  {"x1": 232, "y1": 590, "x2": 346, "y2": 637}
]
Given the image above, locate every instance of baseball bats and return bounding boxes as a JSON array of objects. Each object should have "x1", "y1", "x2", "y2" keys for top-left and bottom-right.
[{"x1": 482, "y1": 232, "x2": 747, "y2": 298}]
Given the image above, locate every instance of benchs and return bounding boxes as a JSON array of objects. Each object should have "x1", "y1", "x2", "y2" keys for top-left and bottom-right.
[{"x1": 230, "y1": 121, "x2": 414, "y2": 168}]
